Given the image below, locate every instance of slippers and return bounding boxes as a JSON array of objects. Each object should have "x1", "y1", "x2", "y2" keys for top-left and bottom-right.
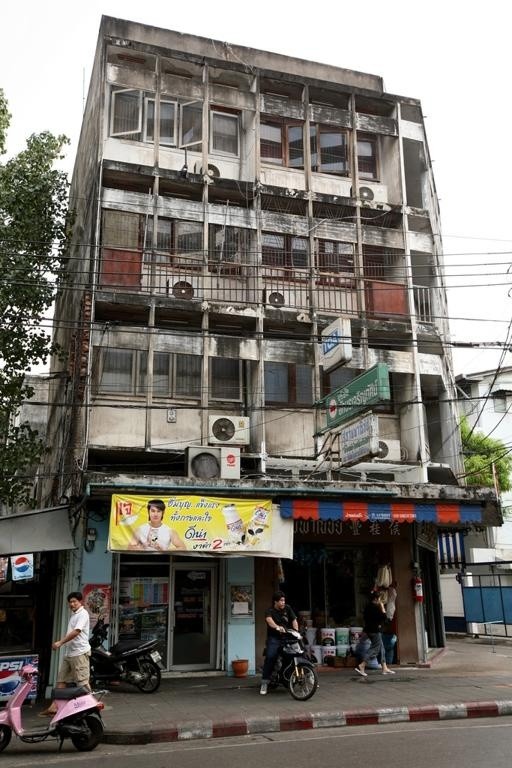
[
  {"x1": 355, "y1": 668, "x2": 368, "y2": 677},
  {"x1": 381, "y1": 670, "x2": 396, "y2": 675}
]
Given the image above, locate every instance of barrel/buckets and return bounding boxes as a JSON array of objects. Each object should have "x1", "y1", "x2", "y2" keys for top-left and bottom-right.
[{"x1": 301, "y1": 611, "x2": 364, "y2": 665}]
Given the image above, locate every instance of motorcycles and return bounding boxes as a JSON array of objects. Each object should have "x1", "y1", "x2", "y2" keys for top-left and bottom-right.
[
  {"x1": 86, "y1": 611, "x2": 166, "y2": 695},
  {"x1": 256, "y1": 622, "x2": 320, "y2": 700},
  {"x1": 0, "y1": 656, "x2": 109, "y2": 755}
]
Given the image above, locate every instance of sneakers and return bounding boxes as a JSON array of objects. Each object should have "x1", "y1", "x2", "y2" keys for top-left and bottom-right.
[
  {"x1": 37, "y1": 709, "x2": 56, "y2": 718},
  {"x1": 259, "y1": 683, "x2": 268, "y2": 695},
  {"x1": 306, "y1": 676, "x2": 320, "y2": 688}
]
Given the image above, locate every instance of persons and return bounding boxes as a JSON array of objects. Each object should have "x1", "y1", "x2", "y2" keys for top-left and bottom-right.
[
  {"x1": 127, "y1": 500, "x2": 186, "y2": 551},
  {"x1": 260, "y1": 591, "x2": 314, "y2": 695},
  {"x1": 38, "y1": 592, "x2": 93, "y2": 718},
  {"x1": 354, "y1": 590, "x2": 396, "y2": 678}
]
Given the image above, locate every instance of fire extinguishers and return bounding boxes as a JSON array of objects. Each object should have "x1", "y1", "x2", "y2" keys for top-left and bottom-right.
[{"x1": 414, "y1": 570, "x2": 424, "y2": 601}]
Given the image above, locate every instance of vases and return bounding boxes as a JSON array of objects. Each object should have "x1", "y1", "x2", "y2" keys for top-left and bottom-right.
[{"x1": 231, "y1": 659, "x2": 249, "y2": 679}]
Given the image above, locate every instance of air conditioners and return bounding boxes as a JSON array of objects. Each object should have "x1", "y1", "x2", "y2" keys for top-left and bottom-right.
[
  {"x1": 184, "y1": 445, "x2": 241, "y2": 479},
  {"x1": 349, "y1": 184, "x2": 380, "y2": 202},
  {"x1": 262, "y1": 287, "x2": 295, "y2": 310},
  {"x1": 373, "y1": 438, "x2": 402, "y2": 463},
  {"x1": 189, "y1": 157, "x2": 223, "y2": 178},
  {"x1": 166, "y1": 278, "x2": 203, "y2": 300},
  {"x1": 208, "y1": 414, "x2": 251, "y2": 445}
]
